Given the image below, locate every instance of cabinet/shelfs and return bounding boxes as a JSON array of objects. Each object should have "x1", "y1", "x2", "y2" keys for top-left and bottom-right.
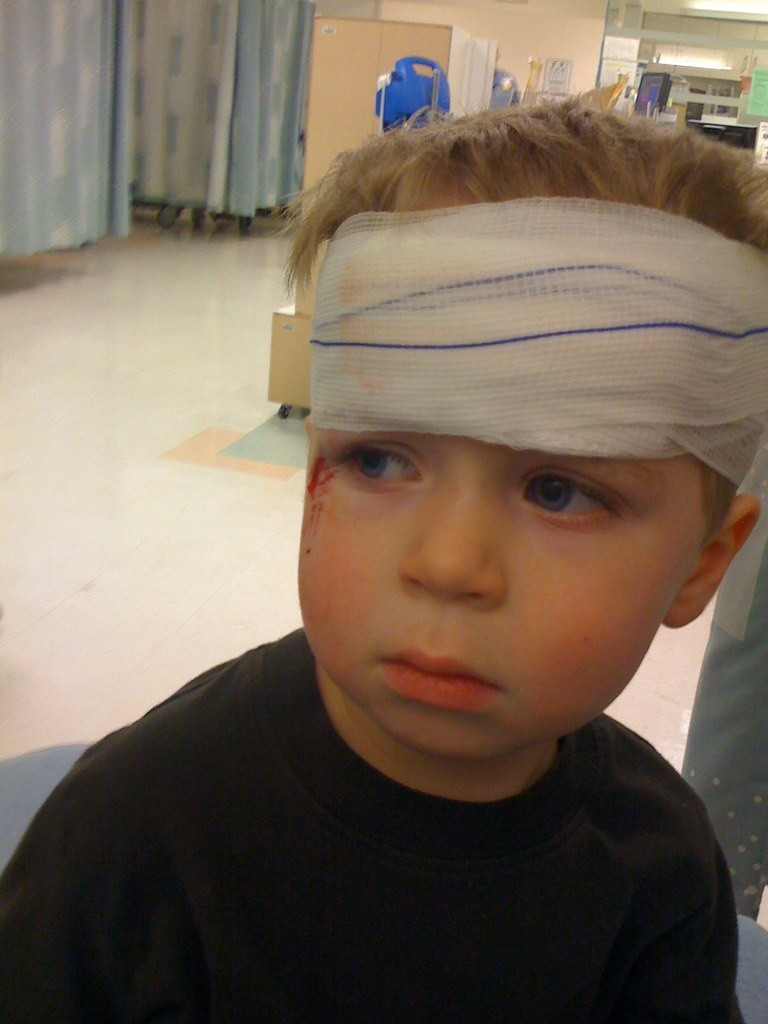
[{"x1": 689, "y1": 119, "x2": 758, "y2": 148}]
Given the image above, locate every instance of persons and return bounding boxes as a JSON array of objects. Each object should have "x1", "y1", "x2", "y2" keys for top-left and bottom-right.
[{"x1": 0, "y1": 97, "x2": 767, "y2": 1024}]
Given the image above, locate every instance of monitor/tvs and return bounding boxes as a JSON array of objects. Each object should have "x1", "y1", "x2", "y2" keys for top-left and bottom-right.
[
  {"x1": 633, "y1": 72, "x2": 673, "y2": 119},
  {"x1": 686, "y1": 120, "x2": 758, "y2": 151}
]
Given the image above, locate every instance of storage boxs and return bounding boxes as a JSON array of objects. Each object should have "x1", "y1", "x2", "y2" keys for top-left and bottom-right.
[
  {"x1": 599, "y1": 61, "x2": 639, "y2": 84},
  {"x1": 601, "y1": 36, "x2": 640, "y2": 60},
  {"x1": 736, "y1": 67, "x2": 768, "y2": 170}
]
